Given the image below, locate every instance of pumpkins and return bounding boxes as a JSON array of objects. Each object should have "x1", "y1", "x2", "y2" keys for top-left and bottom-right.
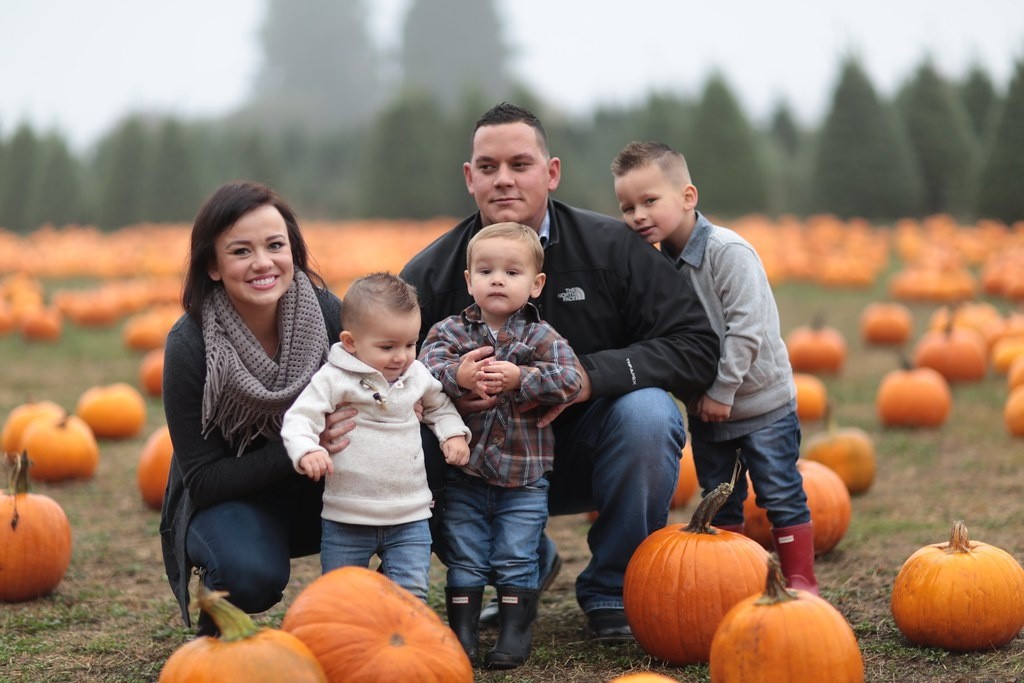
[{"x1": 0, "y1": 217, "x2": 1024, "y2": 683}]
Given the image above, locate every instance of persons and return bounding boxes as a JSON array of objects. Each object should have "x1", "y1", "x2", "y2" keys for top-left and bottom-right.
[
  {"x1": 280, "y1": 272, "x2": 471, "y2": 604},
  {"x1": 399, "y1": 102, "x2": 720, "y2": 647},
  {"x1": 158, "y1": 182, "x2": 424, "y2": 637},
  {"x1": 609, "y1": 141, "x2": 819, "y2": 600},
  {"x1": 418, "y1": 222, "x2": 584, "y2": 670}
]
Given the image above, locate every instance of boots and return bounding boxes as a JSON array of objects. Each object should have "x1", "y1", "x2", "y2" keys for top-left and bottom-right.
[
  {"x1": 442, "y1": 585, "x2": 484, "y2": 664},
  {"x1": 484, "y1": 585, "x2": 540, "y2": 668},
  {"x1": 769, "y1": 520, "x2": 819, "y2": 598}
]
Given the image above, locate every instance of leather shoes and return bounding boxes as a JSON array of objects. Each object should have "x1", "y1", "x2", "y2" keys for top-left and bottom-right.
[
  {"x1": 478, "y1": 549, "x2": 563, "y2": 629},
  {"x1": 588, "y1": 608, "x2": 636, "y2": 642}
]
